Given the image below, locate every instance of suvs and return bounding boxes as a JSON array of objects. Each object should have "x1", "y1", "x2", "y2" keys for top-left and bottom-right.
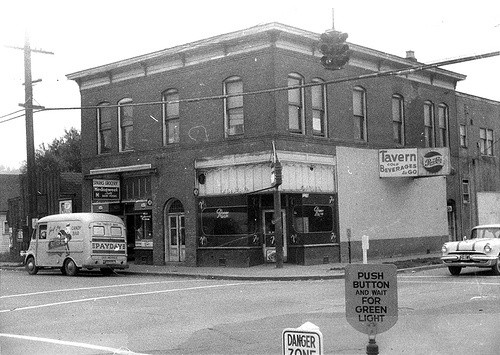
[{"x1": 443, "y1": 225, "x2": 500, "y2": 279}]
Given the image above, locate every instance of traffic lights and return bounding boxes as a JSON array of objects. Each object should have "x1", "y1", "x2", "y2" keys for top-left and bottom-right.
[{"x1": 319, "y1": 29, "x2": 351, "y2": 70}]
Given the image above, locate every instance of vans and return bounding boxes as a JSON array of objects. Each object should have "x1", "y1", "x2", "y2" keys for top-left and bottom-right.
[{"x1": 24, "y1": 211, "x2": 129, "y2": 277}]
